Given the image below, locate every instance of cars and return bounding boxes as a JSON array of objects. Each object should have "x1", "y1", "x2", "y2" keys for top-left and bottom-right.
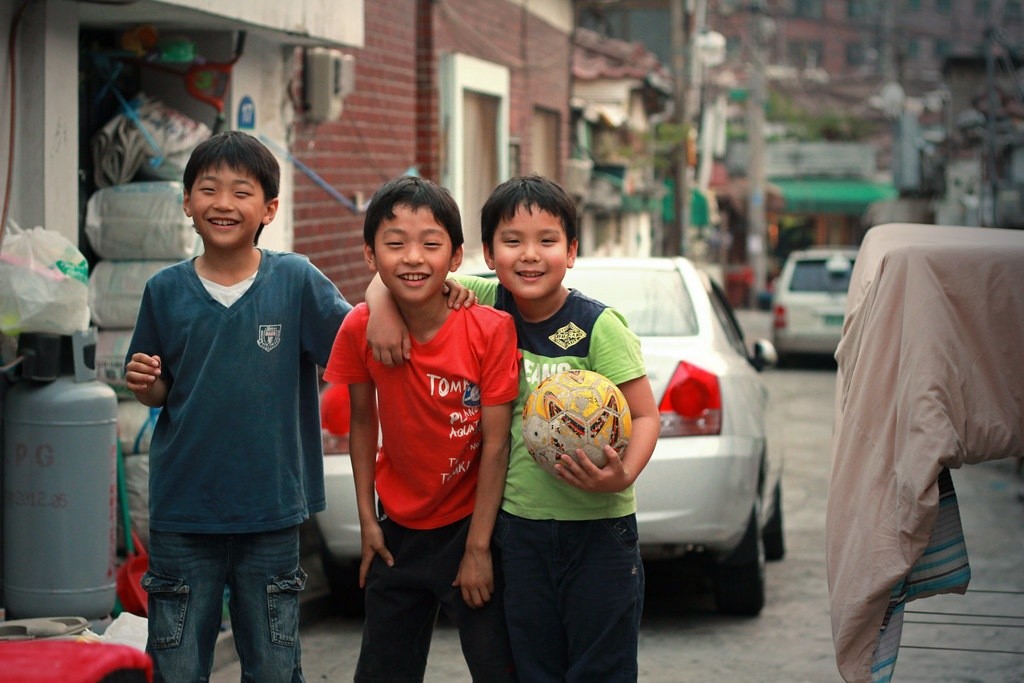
[
  {"x1": 311, "y1": 253, "x2": 786, "y2": 619},
  {"x1": 762, "y1": 247, "x2": 862, "y2": 367}
]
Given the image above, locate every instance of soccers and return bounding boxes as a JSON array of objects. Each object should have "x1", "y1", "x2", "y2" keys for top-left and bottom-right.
[{"x1": 517, "y1": 366, "x2": 636, "y2": 487}]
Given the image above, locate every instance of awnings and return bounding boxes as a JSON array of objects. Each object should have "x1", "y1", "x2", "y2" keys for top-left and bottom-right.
[
  {"x1": 590, "y1": 168, "x2": 722, "y2": 225},
  {"x1": 768, "y1": 177, "x2": 901, "y2": 217}
]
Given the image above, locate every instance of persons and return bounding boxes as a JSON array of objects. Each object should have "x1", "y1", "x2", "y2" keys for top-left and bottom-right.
[
  {"x1": 322, "y1": 176, "x2": 520, "y2": 683},
  {"x1": 364, "y1": 173, "x2": 659, "y2": 683},
  {"x1": 123, "y1": 130, "x2": 478, "y2": 683}
]
